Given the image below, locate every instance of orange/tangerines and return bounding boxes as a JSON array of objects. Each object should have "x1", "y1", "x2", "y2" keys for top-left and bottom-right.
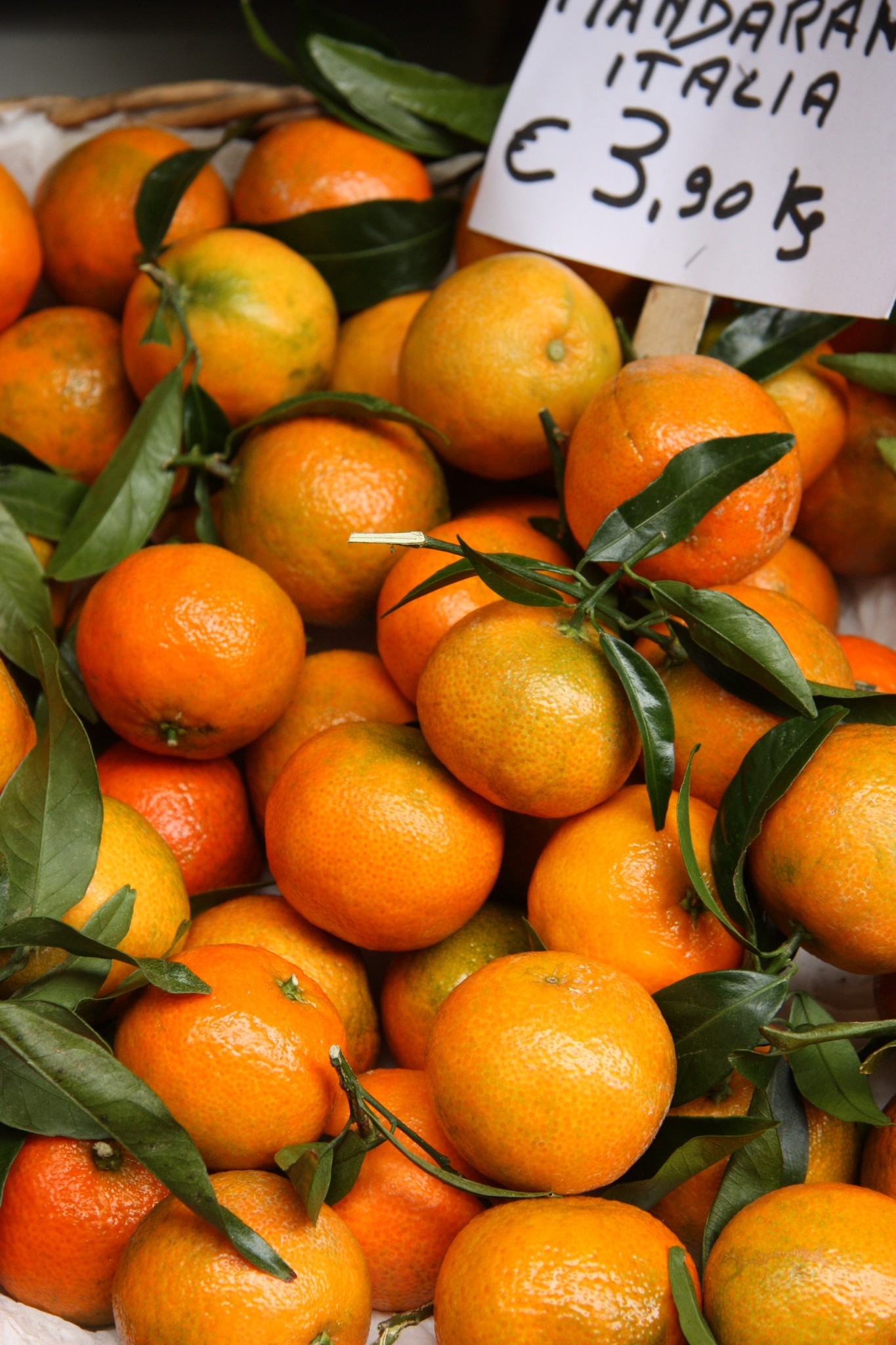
[{"x1": 1, "y1": 117, "x2": 896, "y2": 1344}]
